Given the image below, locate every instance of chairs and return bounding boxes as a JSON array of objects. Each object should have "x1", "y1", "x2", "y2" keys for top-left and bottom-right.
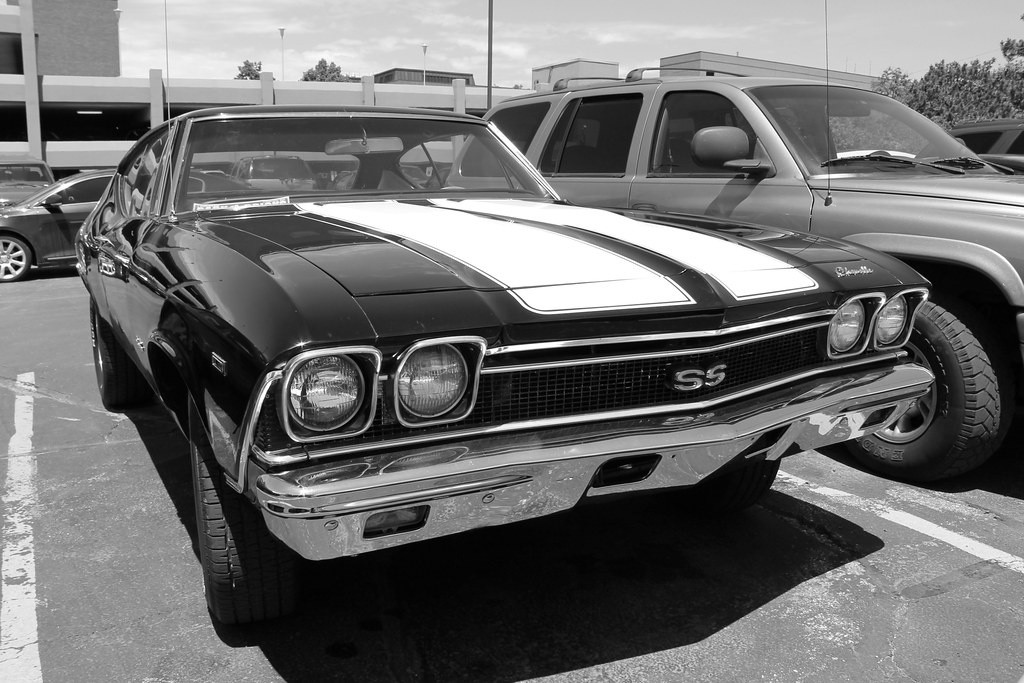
[
  {"x1": 558, "y1": 144, "x2": 618, "y2": 173},
  {"x1": 659, "y1": 137, "x2": 707, "y2": 174},
  {"x1": 27, "y1": 171, "x2": 40, "y2": 181},
  {"x1": 0, "y1": 169, "x2": 14, "y2": 181}
]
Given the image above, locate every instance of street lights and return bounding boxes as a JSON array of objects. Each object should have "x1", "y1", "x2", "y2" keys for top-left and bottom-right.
[
  {"x1": 422, "y1": 44, "x2": 429, "y2": 85},
  {"x1": 279, "y1": 27, "x2": 287, "y2": 81}
]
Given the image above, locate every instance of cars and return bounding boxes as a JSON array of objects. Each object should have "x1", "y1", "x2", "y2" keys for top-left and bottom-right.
[
  {"x1": 1, "y1": 154, "x2": 54, "y2": 206},
  {"x1": 0, "y1": 167, "x2": 256, "y2": 283},
  {"x1": 74, "y1": 105, "x2": 937, "y2": 633}
]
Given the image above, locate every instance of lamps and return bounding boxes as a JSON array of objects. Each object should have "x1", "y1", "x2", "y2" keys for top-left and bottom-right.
[{"x1": 113, "y1": 8, "x2": 124, "y2": 20}]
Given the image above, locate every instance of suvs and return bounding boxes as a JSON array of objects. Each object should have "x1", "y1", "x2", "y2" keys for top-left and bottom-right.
[
  {"x1": 436, "y1": 48, "x2": 1023, "y2": 485},
  {"x1": 233, "y1": 154, "x2": 320, "y2": 193}
]
